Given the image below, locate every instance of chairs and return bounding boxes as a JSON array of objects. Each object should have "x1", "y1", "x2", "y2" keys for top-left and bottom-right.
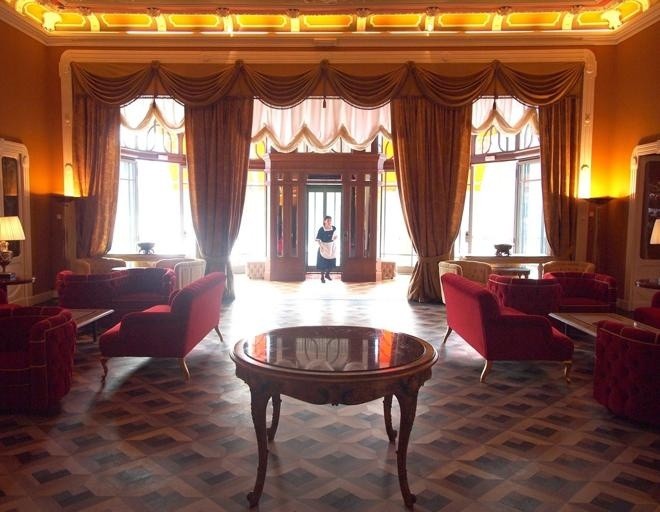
[
  {"x1": 592, "y1": 319, "x2": 659, "y2": 430},
  {"x1": 0, "y1": 301, "x2": 80, "y2": 414},
  {"x1": 432, "y1": 257, "x2": 619, "y2": 384},
  {"x1": 54, "y1": 254, "x2": 207, "y2": 323}
]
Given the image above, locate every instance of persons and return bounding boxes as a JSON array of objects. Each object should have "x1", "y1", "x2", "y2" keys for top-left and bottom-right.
[{"x1": 315, "y1": 214, "x2": 340, "y2": 285}]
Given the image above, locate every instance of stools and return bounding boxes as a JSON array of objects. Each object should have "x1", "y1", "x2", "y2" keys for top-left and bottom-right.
[
  {"x1": 244, "y1": 260, "x2": 265, "y2": 280},
  {"x1": 381, "y1": 261, "x2": 396, "y2": 280}
]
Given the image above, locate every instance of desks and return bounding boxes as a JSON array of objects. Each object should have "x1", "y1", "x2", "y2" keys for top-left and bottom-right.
[
  {"x1": 1, "y1": 272, "x2": 36, "y2": 306},
  {"x1": 226, "y1": 322, "x2": 439, "y2": 509}
]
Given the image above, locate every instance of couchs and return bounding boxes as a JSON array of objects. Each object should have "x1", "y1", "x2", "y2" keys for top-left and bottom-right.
[
  {"x1": 634, "y1": 289, "x2": 660, "y2": 329},
  {"x1": 97, "y1": 269, "x2": 228, "y2": 386}
]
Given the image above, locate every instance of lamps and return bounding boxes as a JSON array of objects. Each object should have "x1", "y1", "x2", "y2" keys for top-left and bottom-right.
[
  {"x1": 579, "y1": 193, "x2": 615, "y2": 272},
  {"x1": 649, "y1": 218, "x2": 660, "y2": 246},
  {"x1": 0, "y1": 214, "x2": 27, "y2": 281}
]
